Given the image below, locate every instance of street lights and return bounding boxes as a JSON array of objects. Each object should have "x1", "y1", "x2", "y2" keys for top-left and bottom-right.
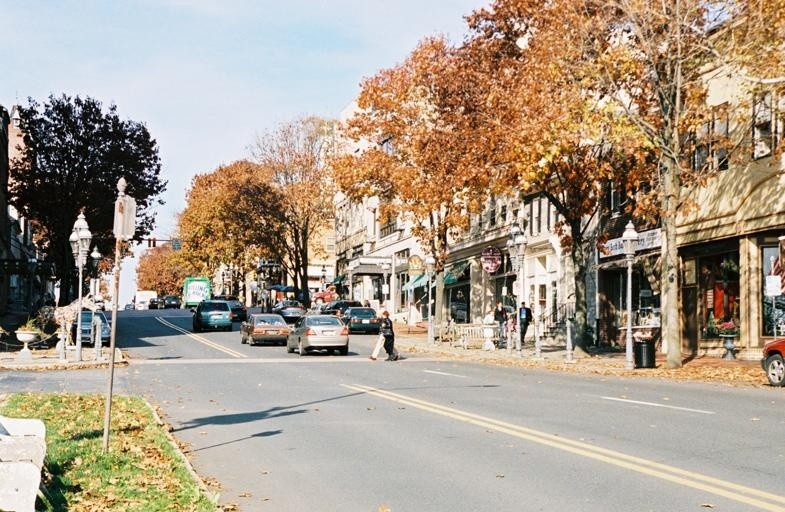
[
  {"x1": 346, "y1": 265, "x2": 353, "y2": 301},
  {"x1": 260, "y1": 257, "x2": 281, "y2": 312},
  {"x1": 505, "y1": 226, "x2": 528, "y2": 326},
  {"x1": 102, "y1": 178, "x2": 136, "y2": 455},
  {"x1": 69, "y1": 206, "x2": 104, "y2": 327},
  {"x1": 224, "y1": 261, "x2": 243, "y2": 295},
  {"x1": 426, "y1": 252, "x2": 435, "y2": 323},
  {"x1": 381, "y1": 261, "x2": 391, "y2": 312},
  {"x1": 622, "y1": 220, "x2": 640, "y2": 333},
  {"x1": 321, "y1": 265, "x2": 326, "y2": 283}
]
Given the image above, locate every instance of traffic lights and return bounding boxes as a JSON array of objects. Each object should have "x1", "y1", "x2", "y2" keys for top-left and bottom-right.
[{"x1": 148, "y1": 238, "x2": 156, "y2": 246}]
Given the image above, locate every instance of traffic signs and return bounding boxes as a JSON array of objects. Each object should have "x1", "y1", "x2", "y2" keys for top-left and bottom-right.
[{"x1": 172, "y1": 239, "x2": 181, "y2": 249}]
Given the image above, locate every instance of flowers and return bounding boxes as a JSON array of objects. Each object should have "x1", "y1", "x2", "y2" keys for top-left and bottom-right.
[{"x1": 719, "y1": 322, "x2": 736, "y2": 335}]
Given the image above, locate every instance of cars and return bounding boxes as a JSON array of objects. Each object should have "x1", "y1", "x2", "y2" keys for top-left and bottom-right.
[
  {"x1": 149, "y1": 295, "x2": 181, "y2": 310},
  {"x1": 321, "y1": 301, "x2": 380, "y2": 334},
  {"x1": 287, "y1": 314, "x2": 349, "y2": 356},
  {"x1": 240, "y1": 313, "x2": 291, "y2": 346},
  {"x1": 272, "y1": 300, "x2": 307, "y2": 321},
  {"x1": 125, "y1": 304, "x2": 134, "y2": 310},
  {"x1": 72, "y1": 311, "x2": 110, "y2": 346},
  {"x1": 191, "y1": 295, "x2": 247, "y2": 332},
  {"x1": 95, "y1": 299, "x2": 105, "y2": 311}
]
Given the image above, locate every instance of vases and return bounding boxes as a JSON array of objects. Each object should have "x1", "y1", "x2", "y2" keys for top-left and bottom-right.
[{"x1": 718, "y1": 334, "x2": 738, "y2": 359}]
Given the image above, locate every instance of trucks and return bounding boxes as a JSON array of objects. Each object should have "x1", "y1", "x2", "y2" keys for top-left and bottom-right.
[
  {"x1": 311, "y1": 285, "x2": 340, "y2": 305},
  {"x1": 183, "y1": 277, "x2": 213, "y2": 309},
  {"x1": 134, "y1": 291, "x2": 158, "y2": 310}
]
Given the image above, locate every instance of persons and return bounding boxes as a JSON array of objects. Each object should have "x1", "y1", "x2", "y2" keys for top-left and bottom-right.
[
  {"x1": 520, "y1": 302, "x2": 533, "y2": 345},
  {"x1": 492, "y1": 301, "x2": 508, "y2": 339},
  {"x1": 370, "y1": 308, "x2": 400, "y2": 361}
]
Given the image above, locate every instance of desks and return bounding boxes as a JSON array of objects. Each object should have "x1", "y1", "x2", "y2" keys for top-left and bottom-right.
[{"x1": 618, "y1": 325, "x2": 660, "y2": 335}]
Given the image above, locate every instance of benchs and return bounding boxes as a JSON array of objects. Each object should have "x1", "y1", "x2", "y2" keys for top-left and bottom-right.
[{"x1": 437, "y1": 324, "x2": 500, "y2": 350}]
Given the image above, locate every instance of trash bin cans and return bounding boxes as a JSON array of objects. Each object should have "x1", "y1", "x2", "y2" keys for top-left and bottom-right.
[{"x1": 633, "y1": 340, "x2": 657, "y2": 369}]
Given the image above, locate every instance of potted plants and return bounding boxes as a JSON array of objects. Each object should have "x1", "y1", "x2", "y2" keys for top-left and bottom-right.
[{"x1": 15, "y1": 314, "x2": 51, "y2": 355}]
